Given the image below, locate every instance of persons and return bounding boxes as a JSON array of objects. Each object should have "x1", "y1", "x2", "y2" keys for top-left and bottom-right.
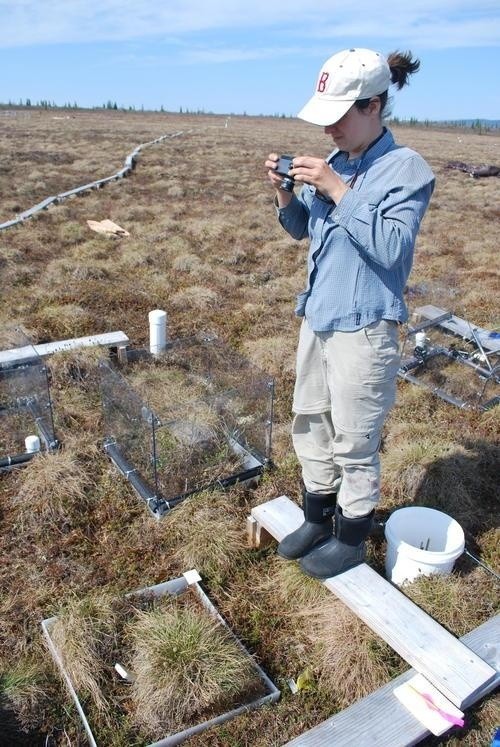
[{"x1": 265, "y1": 43, "x2": 438, "y2": 583}]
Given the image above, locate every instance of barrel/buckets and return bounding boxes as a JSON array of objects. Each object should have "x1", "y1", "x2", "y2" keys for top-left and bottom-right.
[{"x1": 384, "y1": 507, "x2": 467, "y2": 587}]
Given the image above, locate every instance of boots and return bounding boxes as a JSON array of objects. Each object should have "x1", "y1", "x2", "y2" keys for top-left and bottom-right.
[
  {"x1": 278, "y1": 488, "x2": 337, "y2": 559},
  {"x1": 301, "y1": 505, "x2": 375, "y2": 578}
]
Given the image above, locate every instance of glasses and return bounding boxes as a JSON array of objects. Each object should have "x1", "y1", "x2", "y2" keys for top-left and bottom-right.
[{"x1": 314, "y1": 155, "x2": 361, "y2": 205}]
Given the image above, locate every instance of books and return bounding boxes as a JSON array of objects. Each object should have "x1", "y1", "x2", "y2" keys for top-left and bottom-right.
[{"x1": 392, "y1": 670, "x2": 466, "y2": 737}]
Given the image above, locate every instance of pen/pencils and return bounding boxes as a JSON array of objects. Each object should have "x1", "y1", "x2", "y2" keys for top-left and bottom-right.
[{"x1": 405, "y1": 681, "x2": 440, "y2": 711}]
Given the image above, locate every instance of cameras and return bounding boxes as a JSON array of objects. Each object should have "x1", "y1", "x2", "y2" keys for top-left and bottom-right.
[{"x1": 272, "y1": 156, "x2": 307, "y2": 193}]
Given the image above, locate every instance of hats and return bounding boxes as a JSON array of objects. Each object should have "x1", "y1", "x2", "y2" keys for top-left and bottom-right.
[{"x1": 298, "y1": 49, "x2": 390, "y2": 127}]
